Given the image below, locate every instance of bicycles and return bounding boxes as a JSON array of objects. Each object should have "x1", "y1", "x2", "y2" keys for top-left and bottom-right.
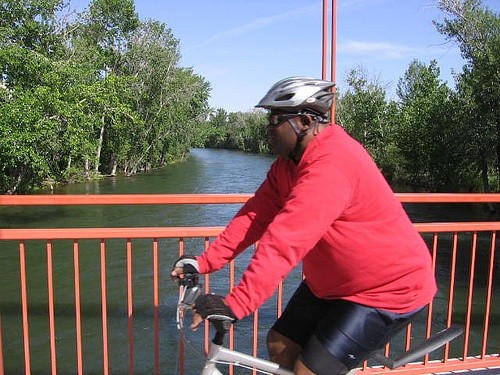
[{"x1": 172, "y1": 259, "x2": 464, "y2": 374}]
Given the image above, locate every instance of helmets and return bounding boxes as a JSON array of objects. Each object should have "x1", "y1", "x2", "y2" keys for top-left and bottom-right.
[{"x1": 254, "y1": 75, "x2": 336, "y2": 119}]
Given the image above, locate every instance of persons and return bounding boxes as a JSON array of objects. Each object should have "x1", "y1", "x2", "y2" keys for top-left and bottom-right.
[{"x1": 168, "y1": 74, "x2": 438, "y2": 375}]
